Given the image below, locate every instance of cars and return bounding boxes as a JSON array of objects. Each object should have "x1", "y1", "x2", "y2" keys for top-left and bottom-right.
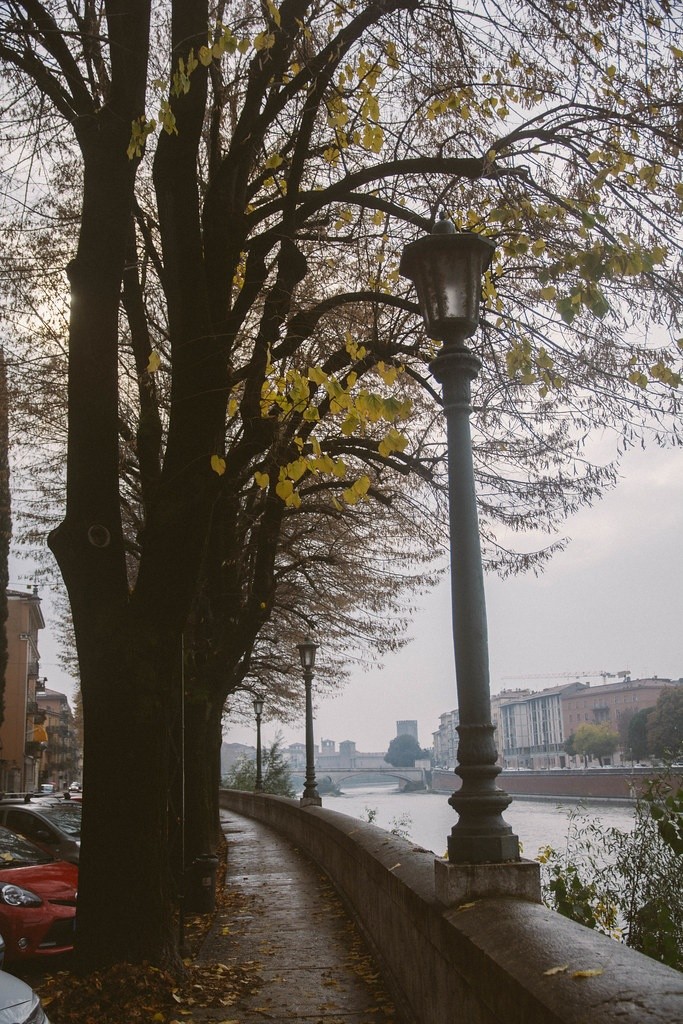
[
  {"x1": 39, "y1": 783, "x2": 55, "y2": 794},
  {"x1": 0, "y1": 789, "x2": 82, "y2": 866},
  {"x1": 0, "y1": 969, "x2": 51, "y2": 1024},
  {"x1": 69, "y1": 782, "x2": 82, "y2": 793},
  {"x1": 0, "y1": 824, "x2": 82, "y2": 963}
]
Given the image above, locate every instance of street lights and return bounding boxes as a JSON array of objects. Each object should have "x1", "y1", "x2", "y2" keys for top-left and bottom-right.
[
  {"x1": 251, "y1": 693, "x2": 264, "y2": 793},
  {"x1": 395, "y1": 208, "x2": 543, "y2": 901},
  {"x1": 295, "y1": 633, "x2": 323, "y2": 807}
]
